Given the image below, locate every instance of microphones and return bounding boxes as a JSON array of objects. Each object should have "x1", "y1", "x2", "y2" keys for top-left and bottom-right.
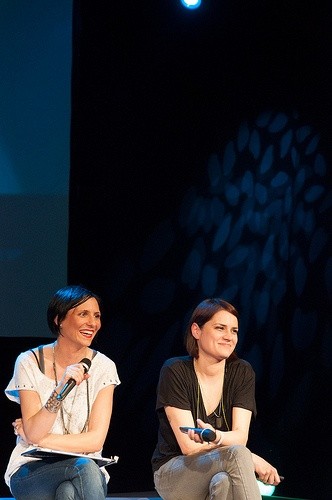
[
  {"x1": 56, "y1": 358, "x2": 91, "y2": 401},
  {"x1": 180, "y1": 427, "x2": 216, "y2": 442}
]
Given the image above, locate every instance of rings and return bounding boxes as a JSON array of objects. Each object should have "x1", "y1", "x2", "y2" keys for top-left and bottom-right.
[{"x1": 15, "y1": 424, "x2": 20, "y2": 428}]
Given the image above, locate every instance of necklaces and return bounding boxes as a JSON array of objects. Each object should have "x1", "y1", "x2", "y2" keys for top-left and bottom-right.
[
  {"x1": 194, "y1": 358, "x2": 222, "y2": 429},
  {"x1": 53, "y1": 340, "x2": 90, "y2": 434}
]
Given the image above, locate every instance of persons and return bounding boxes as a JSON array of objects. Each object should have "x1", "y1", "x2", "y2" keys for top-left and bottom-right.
[
  {"x1": 3, "y1": 284, "x2": 121, "y2": 500},
  {"x1": 151, "y1": 298, "x2": 280, "y2": 500}
]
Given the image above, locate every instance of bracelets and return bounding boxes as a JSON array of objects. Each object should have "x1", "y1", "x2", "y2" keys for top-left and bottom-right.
[
  {"x1": 217, "y1": 431, "x2": 224, "y2": 445},
  {"x1": 43, "y1": 389, "x2": 61, "y2": 414}
]
